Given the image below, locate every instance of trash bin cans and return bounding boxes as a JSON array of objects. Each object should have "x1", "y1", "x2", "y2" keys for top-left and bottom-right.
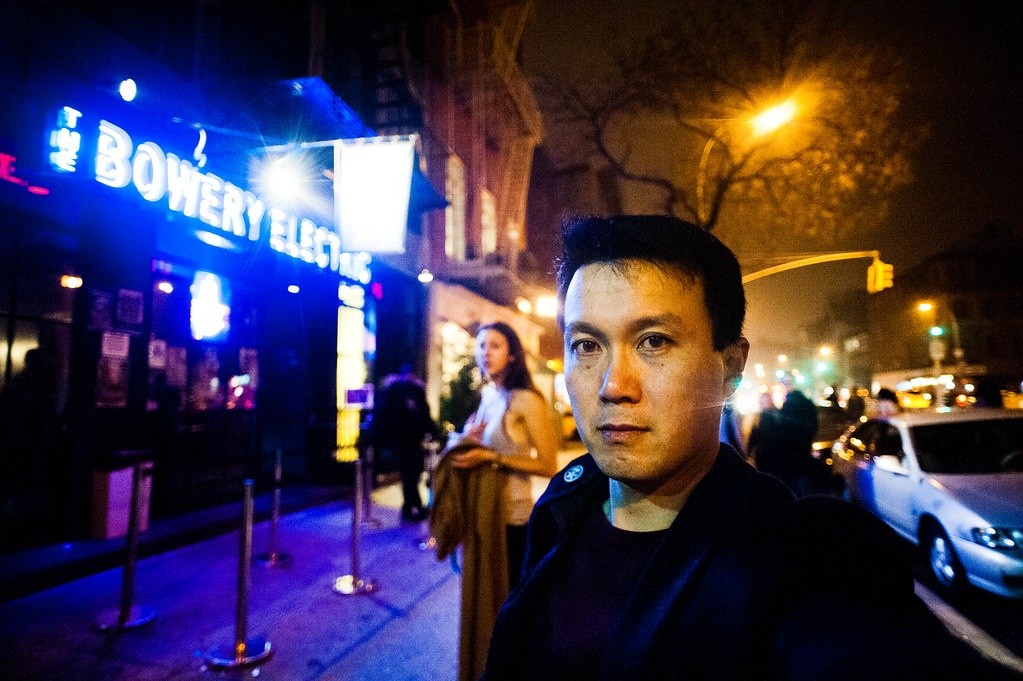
[{"x1": 91, "y1": 452, "x2": 154, "y2": 541}]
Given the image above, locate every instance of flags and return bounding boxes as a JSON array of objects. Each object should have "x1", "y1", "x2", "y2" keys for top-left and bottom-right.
[{"x1": 334, "y1": 134, "x2": 415, "y2": 254}]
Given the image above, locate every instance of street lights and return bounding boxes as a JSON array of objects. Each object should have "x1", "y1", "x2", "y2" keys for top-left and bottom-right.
[
  {"x1": 696, "y1": 99, "x2": 796, "y2": 223},
  {"x1": 914, "y1": 296, "x2": 962, "y2": 351}
]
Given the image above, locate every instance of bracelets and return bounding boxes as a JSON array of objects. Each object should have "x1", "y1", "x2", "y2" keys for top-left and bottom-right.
[{"x1": 491, "y1": 450, "x2": 500, "y2": 471}]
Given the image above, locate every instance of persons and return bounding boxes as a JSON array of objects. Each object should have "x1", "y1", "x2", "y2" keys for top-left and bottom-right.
[
  {"x1": 353, "y1": 359, "x2": 449, "y2": 522},
  {"x1": 718, "y1": 374, "x2": 904, "y2": 496},
  {"x1": 446, "y1": 320, "x2": 558, "y2": 681},
  {"x1": 481, "y1": 214, "x2": 915, "y2": 680}
]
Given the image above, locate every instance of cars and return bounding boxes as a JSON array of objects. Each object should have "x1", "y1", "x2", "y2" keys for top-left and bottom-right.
[{"x1": 829, "y1": 409, "x2": 1023, "y2": 600}]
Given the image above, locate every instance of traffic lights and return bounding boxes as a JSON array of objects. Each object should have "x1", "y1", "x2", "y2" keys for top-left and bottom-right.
[
  {"x1": 864, "y1": 263, "x2": 895, "y2": 295},
  {"x1": 930, "y1": 327, "x2": 945, "y2": 337}
]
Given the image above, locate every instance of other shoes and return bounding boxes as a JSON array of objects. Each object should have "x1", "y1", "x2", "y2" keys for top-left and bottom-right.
[
  {"x1": 419, "y1": 505, "x2": 428, "y2": 518},
  {"x1": 402, "y1": 506, "x2": 413, "y2": 519}
]
